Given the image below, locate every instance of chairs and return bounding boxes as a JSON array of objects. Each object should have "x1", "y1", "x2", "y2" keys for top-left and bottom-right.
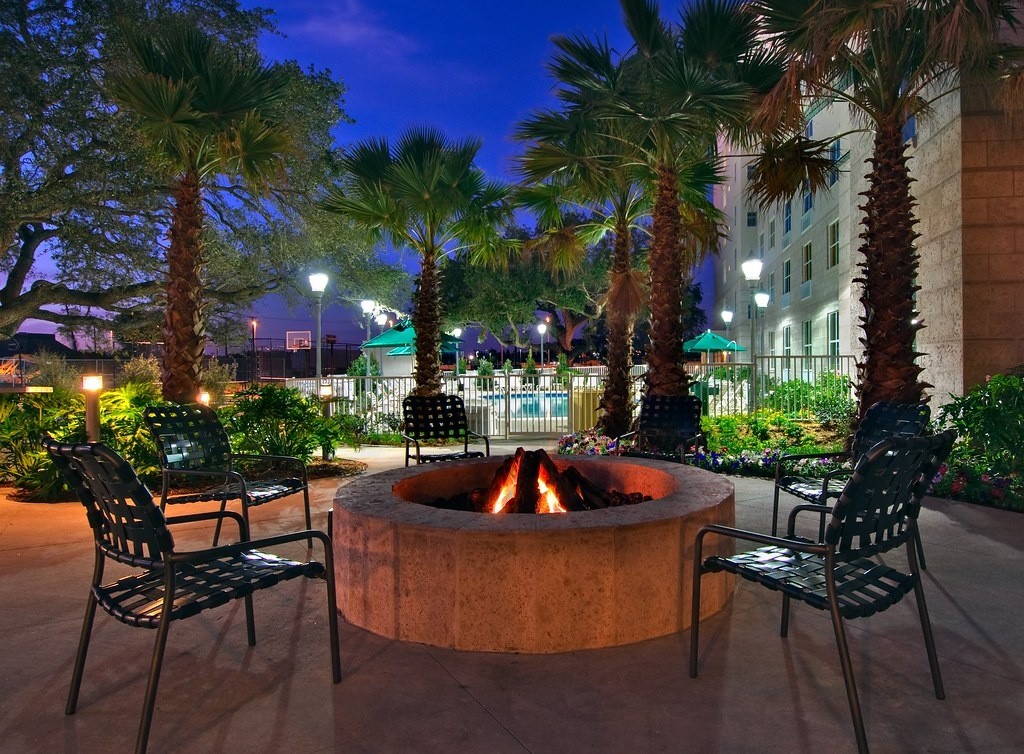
[
  {"x1": 394, "y1": 380, "x2": 404, "y2": 389},
  {"x1": 536, "y1": 373, "x2": 554, "y2": 392},
  {"x1": 687, "y1": 428, "x2": 958, "y2": 754},
  {"x1": 573, "y1": 375, "x2": 584, "y2": 390},
  {"x1": 383, "y1": 380, "x2": 404, "y2": 396},
  {"x1": 402, "y1": 394, "x2": 490, "y2": 467},
  {"x1": 771, "y1": 400, "x2": 931, "y2": 571},
  {"x1": 496, "y1": 374, "x2": 523, "y2": 391},
  {"x1": 143, "y1": 403, "x2": 314, "y2": 549},
  {"x1": 458, "y1": 374, "x2": 481, "y2": 391},
  {"x1": 373, "y1": 381, "x2": 404, "y2": 406},
  {"x1": 585, "y1": 372, "x2": 598, "y2": 389},
  {"x1": 709, "y1": 382, "x2": 731, "y2": 413},
  {"x1": 40, "y1": 436, "x2": 342, "y2": 754},
  {"x1": 367, "y1": 391, "x2": 398, "y2": 414},
  {"x1": 615, "y1": 394, "x2": 704, "y2": 466},
  {"x1": 723, "y1": 379, "x2": 748, "y2": 410},
  {"x1": 696, "y1": 374, "x2": 704, "y2": 382}
]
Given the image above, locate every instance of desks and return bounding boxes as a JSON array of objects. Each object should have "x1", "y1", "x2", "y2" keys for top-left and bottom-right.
[
  {"x1": 553, "y1": 383, "x2": 562, "y2": 391},
  {"x1": 523, "y1": 383, "x2": 535, "y2": 391}
]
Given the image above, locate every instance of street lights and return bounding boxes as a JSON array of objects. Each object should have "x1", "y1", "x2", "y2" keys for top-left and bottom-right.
[
  {"x1": 452, "y1": 327, "x2": 462, "y2": 379},
  {"x1": 307, "y1": 273, "x2": 330, "y2": 400},
  {"x1": 742, "y1": 257, "x2": 800, "y2": 412},
  {"x1": 538, "y1": 323, "x2": 546, "y2": 373},
  {"x1": 374, "y1": 314, "x2": 388, "y2": 377},
  {"x1": 722, "y1": 308, "x2": 734, "y2": 381},
  {"x1": 753, "y1": 282, "x2": 771, "y2": 396},
  {"x1": 360, "y1": 298, "x2": 375, "y2": 398}
]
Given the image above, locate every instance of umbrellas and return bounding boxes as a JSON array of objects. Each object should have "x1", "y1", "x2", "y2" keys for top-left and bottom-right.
[
  {"x1": 357, "y1": 314, "x2": 465, "y2": 375},
  {"x1": 683, "y1": 329, "x2": 749, "y2": 364}
]
